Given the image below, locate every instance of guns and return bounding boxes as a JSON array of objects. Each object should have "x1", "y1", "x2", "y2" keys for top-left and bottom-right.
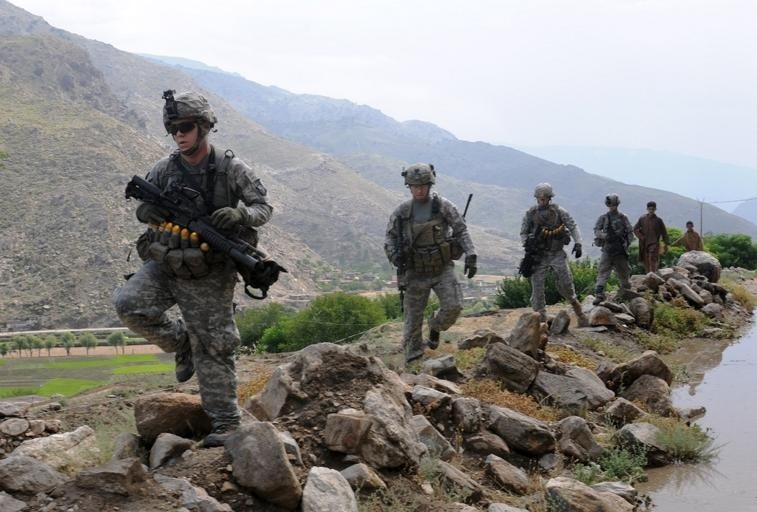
[
  {"x1": 396, "y1": 215, "x2": 406, "y2": 313},
  {"x1": 519, "y1": 221, "x2": 547, "y2": 277},
  {"x1": 125, "y1": 175, "x2": 288, "y2": 289},
  {"x1": 605, "y1": 224, "x2": 629, "y2": 261}
]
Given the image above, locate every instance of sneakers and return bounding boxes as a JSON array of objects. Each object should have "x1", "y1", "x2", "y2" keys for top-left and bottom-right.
[
  {"x1": 592, "y1": 293, "x2": 606, "y2": 306},
  {"x1": 172, "y1": 316, "x2": 198, "y2": 383},
  {"x1": 203, "y1": 426, "x2": 238, "y2": 446},
  {"x1": 427, "y1": 329, "x2": 441, "y2": 350}
]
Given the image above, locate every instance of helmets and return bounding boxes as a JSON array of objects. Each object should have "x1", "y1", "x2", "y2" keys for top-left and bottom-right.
[
  {"x1": 402, "y1": 162, "x2": 436, "y2": 187},
  {"x1": 605, "y1": 193, "x2": 620, "y2": 207},
  {"x1": 533, "y1": 182, "x2": 555, "y2": 198},
  {"x1": 647, "y1": 201, "x2": 656, "y2": 207},
  {"x1": 162, "y1": 90, "x2": 218, "y2": 128}
]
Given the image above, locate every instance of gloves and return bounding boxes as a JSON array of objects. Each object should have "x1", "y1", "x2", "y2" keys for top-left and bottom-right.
[
  {"x1": 210, "y1": 205, "x2": 242, "y2": 229},
  {"x1": 463, "y1": 253, "x2": 478, "y2": 279},
  {"x1": 135, "y1": 201, "x2": 154, "y2": 224},
  {"x1": 571, "y1": 243, "x2": 582, "y2": 258}
]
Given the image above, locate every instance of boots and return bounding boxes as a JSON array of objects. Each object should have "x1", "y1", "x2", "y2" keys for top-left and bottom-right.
[{"x1": 571, "y1": 300, "x2": 584, "y2": 316}]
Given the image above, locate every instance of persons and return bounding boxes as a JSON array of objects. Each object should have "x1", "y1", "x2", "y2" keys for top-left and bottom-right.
[
  {"x1": 520, "y1": 181, "x2": 586, "y2": 321},
  {"x1": 682, "y1": 222, "x2": 702, "y2": 251},
  {"x1": 633, "y1": 200, "x2": 668, "y2": 274},
  {"x1": 383, "y1": 161, "x2": 477, "y2": 370},
  {"x1": 112, "y1": 94, "x2": 274, "y2": 449},
  {"x1": 592, "y1": 193, "x2": 633, "y2": 304}
]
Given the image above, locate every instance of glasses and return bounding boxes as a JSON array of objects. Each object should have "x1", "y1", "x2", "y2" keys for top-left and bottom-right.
[{"x1": 171, "y1": 120, "x2": 197, "y2": 135}]
